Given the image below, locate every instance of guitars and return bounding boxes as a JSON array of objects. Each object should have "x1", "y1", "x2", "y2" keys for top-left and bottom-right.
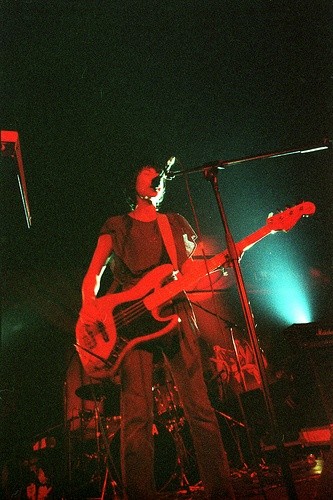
[{"x1": 76, "y1": 200, "x2": 317, "y2": 380}]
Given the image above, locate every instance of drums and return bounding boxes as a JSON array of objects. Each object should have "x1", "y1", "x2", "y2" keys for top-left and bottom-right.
[
  {"x1": 106, "y1": 419, "x2": 177, "y2": 492},
  {"x1": 63, "y1": 428, "x2": 105, "y2": 479}
]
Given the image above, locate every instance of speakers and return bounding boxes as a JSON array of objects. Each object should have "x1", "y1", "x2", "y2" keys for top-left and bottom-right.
[{"x1": 283, "y1": 321, "x2": 333, "y2": 425}]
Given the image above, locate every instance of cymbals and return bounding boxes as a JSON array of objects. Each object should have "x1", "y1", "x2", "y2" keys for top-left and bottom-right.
[
  {"x1": 74, "y1": 383, "x2": 102, "y2": 401},
  {"x1": 149, "y1": 365, "x2": 167, "y2": 385},
  {"x1": 157, "y1": 406, "x2": 183, "y2": 424}
]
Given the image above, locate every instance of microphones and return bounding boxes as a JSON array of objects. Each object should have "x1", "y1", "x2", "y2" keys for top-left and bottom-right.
[{"x1": 150, "y1": 156, "x2": 179, "y2": 190}]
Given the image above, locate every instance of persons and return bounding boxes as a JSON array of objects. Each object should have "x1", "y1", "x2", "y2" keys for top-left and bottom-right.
[{"x1": 76, "y1": 161, "x2": 239, "y2": 500}]
